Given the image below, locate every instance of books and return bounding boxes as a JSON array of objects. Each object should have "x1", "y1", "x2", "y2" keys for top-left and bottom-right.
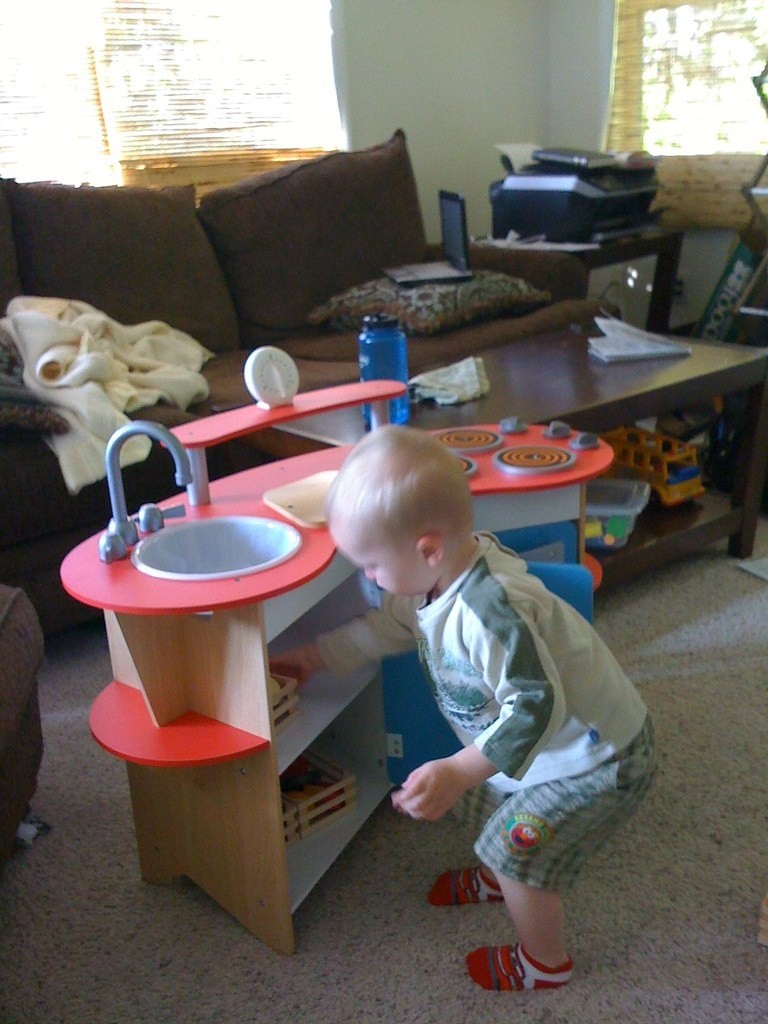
[{"x1": 585, "y1": 304, "x2": 691, "y2": 365}]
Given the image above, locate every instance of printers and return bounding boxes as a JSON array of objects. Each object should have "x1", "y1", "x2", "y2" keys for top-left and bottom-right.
[{"x1": 490, "y1": 147, "x2": 666, "y2": 242}]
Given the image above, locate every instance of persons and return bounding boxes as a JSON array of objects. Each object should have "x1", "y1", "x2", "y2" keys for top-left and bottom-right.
[{"x1": 270, "y1": 424, "x2": 660, "y2": 997}]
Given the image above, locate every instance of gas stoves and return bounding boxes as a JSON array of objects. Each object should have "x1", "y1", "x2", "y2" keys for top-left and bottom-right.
[{"x1": 421, "y1": 417, "x2": 615, "y2": 491}]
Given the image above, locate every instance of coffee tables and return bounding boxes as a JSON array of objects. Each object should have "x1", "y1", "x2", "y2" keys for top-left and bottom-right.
[{"x1": 211, "y1": 313, "x2": 768, "y2": 599}]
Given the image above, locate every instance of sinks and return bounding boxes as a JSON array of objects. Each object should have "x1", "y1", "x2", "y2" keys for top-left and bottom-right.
[{"x1": 130, "y1": 515, "x2": 303, "y2": 581}]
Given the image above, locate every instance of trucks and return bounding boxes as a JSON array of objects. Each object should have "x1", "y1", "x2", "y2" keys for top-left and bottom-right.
[{"x1": 604, "y1": 425, "x2": 707, "y2": 515}]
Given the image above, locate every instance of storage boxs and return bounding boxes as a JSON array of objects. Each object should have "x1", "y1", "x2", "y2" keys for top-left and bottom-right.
[
  {"x1": 279, "y1": 748, "x2": 358, "y2": 845},
  {"x1": 585, "y1": 478, "x2": 651, "y2": 553},
  {"x1": 269, "y1": 673, "x2": 301, "y2": 736}
]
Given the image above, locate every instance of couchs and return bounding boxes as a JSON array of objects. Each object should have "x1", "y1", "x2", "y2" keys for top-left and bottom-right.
[
  {"x1": 0, "y1": 179, "x2": 622, "y2": 652},
  {"x1": 0, "y1": 583, "x2": 47, "y2": 872}
]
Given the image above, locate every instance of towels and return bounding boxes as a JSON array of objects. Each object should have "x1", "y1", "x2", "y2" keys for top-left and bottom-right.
[{"x1": 0, "y1": 297, "x2": 214, "y2": 495}]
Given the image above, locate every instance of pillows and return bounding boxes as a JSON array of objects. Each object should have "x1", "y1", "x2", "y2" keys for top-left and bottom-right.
[
  {"x1": 3, "y1": 178, "x2": 243, "y2": 353},
  {"x1": 200, "y1": 129, "x2": 428, "y2": 351},
  {"x1": 0, "y1": 178, "x2": 25, "y2": 318},
  {"x1": 306, "y1": 265, "x2": 551, "y2": 339},
  {"x1": 0, "y1": 327, "x2": 69, "y2": 435}
]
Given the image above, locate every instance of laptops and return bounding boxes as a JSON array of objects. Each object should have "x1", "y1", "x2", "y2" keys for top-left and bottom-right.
[{"x1": 378, "y1": 190, "x2": 474, "y2": 284}]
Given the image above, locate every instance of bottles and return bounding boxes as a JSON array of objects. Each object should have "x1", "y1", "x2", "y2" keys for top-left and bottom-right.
[{"x1": 357, "y1": 313, "x2": 409, "y2": 424}]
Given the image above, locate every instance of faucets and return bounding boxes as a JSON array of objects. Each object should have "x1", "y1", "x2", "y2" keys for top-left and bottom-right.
[{"x1": 105, "y1": 420, "x2": 193, "y2": 546}]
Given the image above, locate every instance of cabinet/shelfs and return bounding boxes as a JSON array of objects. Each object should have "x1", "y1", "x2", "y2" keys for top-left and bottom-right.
[
  {"x1": 58, "y1": 379, "x2": 614, "y2": 958},
  {"x1": 425, "y1": 222, "x2": 683, "y2": 337}
]
